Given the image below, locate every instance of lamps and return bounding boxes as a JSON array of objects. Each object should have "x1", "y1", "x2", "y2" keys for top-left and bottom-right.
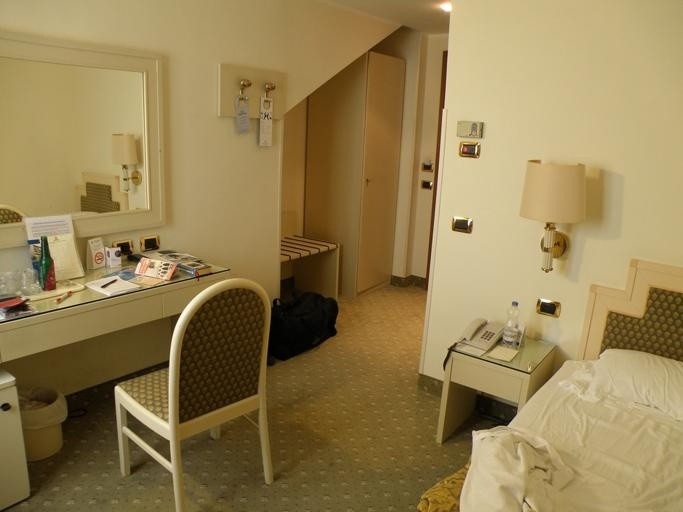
[
  {"x1": 112, "y1": 134, "x2": 142, "y2": 192},
  {"x1": 519, "y1": 159, "x2": 586, "y2": 273}
]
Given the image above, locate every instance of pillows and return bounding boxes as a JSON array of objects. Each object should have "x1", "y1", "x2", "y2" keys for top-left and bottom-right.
[{"x1": 586, "y1": 349, "x2": 683, "y2": 422}]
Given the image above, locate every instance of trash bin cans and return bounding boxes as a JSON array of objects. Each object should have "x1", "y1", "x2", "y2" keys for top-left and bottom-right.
[{"x1": 17, "y1": 385, "x2": 65, "y2": 463}]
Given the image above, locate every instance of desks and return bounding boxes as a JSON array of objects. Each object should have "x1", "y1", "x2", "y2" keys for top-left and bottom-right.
[{"x1": 0, "y1": 249, "x2": 231, "y2": 396}]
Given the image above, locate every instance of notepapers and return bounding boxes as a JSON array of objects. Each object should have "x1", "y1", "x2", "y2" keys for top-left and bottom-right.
[{"x1": 486, "y1": 344, "x2": 520, "y2": 363}]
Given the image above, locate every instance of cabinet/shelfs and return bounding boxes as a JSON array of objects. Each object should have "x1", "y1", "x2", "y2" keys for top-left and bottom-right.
[
  {"x1": 303, "y1": 52, "x2": 406, "y2": 301},
  {"x1": 0, "y1": 368, "x2": 30, "y2": 511}
]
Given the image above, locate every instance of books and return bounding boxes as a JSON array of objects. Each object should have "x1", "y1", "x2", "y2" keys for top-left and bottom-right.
[{"x1": 85, "y1": 275, "x2": 140, "y2": 298}]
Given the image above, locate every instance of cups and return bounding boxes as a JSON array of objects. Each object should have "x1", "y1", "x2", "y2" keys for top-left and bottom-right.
[
  {"x1": 1, "y1": 268, "x2": 20, "y2": 293},
  {"x1": 20, "y1": 268, "x2": 40, "y2": 295}
]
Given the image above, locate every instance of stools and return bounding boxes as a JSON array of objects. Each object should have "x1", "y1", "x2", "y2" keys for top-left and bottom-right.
[{"x1": 280, "y1": 235, "x2": 340, "y2": 301}]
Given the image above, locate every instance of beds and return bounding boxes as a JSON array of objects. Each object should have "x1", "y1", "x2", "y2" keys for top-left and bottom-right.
[
  {"x1": 70, "y1": 171, "x2": 129, "y2": 218},
  {"x1": 417, "y1": 258, "x2": 683, "y2": 512}
]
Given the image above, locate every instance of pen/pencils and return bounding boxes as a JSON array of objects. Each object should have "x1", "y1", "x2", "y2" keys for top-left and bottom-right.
[
  {"x1": 55, "y1": 291, "x2": 71, "y2": 302},
  {"x1": 101, "y1": 279, "x2": 117, "y2": 288}
]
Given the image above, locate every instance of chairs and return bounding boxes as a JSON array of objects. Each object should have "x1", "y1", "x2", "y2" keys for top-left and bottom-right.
[
  {"x1": 114, "y1": 278, "x2": 274, "y2": 512},
  {"x1": 0, "y1": 203, "x2": 26, "y2": 224}
]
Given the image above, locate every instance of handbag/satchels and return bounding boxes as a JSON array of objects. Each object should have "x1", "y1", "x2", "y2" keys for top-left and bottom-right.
[{"x1": 268, "y1": 291, "x2": 338, "y2": 360}]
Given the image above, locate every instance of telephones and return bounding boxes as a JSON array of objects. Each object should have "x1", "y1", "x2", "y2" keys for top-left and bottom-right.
[{"x1": 457, "y1": 317, "x2": 505, "y2": 351}]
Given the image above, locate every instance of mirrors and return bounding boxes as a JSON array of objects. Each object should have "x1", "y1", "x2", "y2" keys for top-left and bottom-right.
[{"x1": 0, "y1": 29, "x2": 165, "y2": 249}]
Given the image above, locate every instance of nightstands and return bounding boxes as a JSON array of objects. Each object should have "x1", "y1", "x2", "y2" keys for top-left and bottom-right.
[{"x1": 436, "y1": 335, "x2": 559, "y2": 445}]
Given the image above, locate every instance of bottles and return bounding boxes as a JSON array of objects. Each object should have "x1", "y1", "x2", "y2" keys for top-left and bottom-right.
[
  {"x1": 502, "y1": 301, "x2": 520, "y2": 347},
  {"x1": 37, "y1": 236, "x2": 56, "y2": 291}
]
